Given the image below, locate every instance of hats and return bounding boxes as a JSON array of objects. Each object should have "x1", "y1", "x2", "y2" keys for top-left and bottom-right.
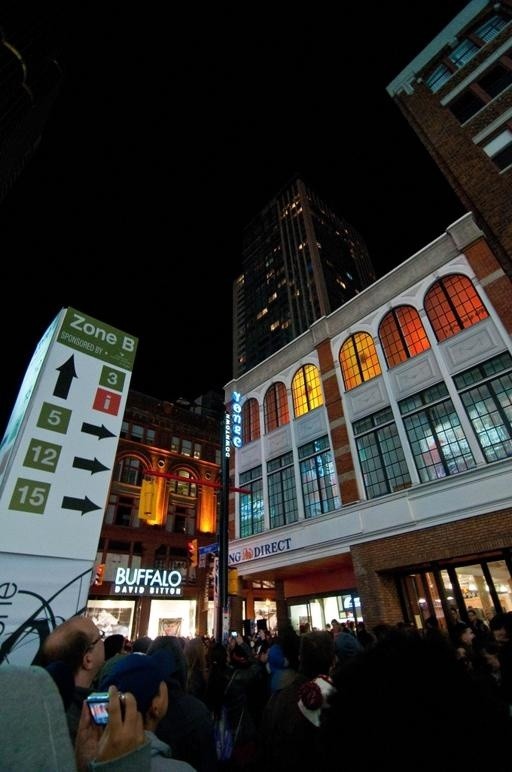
[{"x1": 101, "y1": 648, "x2": 175, "y2": 707}]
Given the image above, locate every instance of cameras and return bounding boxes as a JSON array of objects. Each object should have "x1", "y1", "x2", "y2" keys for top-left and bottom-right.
[{"x1": 86, "y1": 692, "x2": 125, "y2": 725}]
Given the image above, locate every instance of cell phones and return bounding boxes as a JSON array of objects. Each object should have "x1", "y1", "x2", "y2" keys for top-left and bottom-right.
[{"x1": 230, "y1": 631, "x2": 237, "y2": 639}]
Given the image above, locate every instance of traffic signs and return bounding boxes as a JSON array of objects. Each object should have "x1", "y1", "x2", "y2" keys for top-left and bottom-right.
[{"x1": 199, "y1": 543, "x2": 219, "y2": 556}]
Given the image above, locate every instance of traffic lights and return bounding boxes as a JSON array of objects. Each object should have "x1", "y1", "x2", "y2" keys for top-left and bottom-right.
[
  {"x1": 95, "y1": 564, "x2": 106, "y2": 586},
  {"x1": 188, "y1": 538, "x2": 198, "y2": 569}
]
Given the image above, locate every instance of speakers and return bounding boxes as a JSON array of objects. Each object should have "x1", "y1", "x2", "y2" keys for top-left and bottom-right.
[
  {"x1": 257, "y1": 618, "x2": 266, "y2": 632},
  {"x1": 244, "y1": 619, "x2": 255, "y2": 635}
]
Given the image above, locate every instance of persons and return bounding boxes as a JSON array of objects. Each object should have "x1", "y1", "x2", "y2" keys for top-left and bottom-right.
[{"x1": 1, "y1": 606, "x2": 510, "y2": 769}]
